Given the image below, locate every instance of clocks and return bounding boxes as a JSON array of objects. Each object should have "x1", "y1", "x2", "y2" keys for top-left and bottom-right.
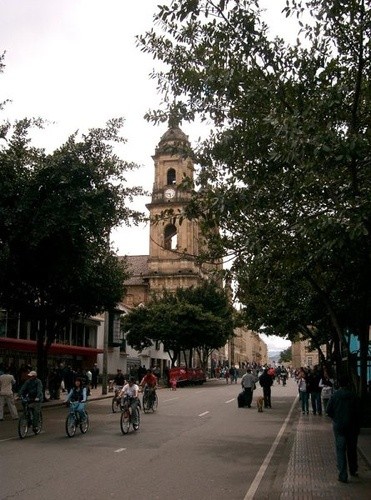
[{"x1": 164, "y1": 188, "x2": 176, "y2": 199}]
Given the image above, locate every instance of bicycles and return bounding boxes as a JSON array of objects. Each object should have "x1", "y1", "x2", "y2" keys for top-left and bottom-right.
[
  {"x1": 16, "y1": 396, "x2": 43, "y2": 439},
  {"x1": 111, "y1": 389, "x2": 120, "y2": 413},
  {"x1": 140, "y1": 387, "x2": 159, "y2": 415},
  {"x1": 120, "y1": 394, "x2": 141, "y2": 434},
  {"x1": 66, "y1": 401, "x2": 90, "y2": 437}
]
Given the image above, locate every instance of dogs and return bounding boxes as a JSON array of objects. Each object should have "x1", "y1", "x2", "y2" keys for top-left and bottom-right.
[{"x1": 255, "y1": 396, "x2": 265, "y2": 413}]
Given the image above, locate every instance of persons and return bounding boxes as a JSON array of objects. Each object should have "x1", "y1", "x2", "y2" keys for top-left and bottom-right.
[
  {"x1": 56, "y1": 362, "x2": 334, "y2": 416},
  {"x1": 115, "y1": 377, "x2": 140, "y2": 425},
  {"x1": 325, "y1": 373, "x2": 366, "y2": 483},
  {"x1": 16, "y1": 370, "x2": 42, "y2": 431},
  {"x1": 0, "y1": 364, "x2": 20, "y2": 421},
  {"x1": 64, "y1": 378, "x2": 88, "y2": 425}
]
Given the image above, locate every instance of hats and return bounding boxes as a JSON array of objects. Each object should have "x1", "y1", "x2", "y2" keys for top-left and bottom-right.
[{"x1": 27, "y1": 371, "x2": 37, "y2": 376}]
